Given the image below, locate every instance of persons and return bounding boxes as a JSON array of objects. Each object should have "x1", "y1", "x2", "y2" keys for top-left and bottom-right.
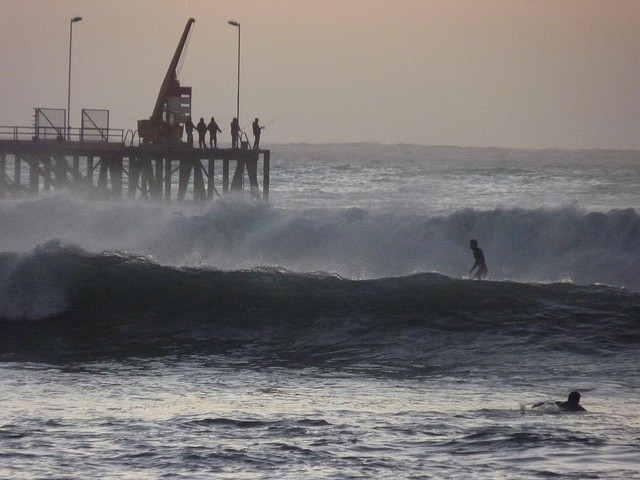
[
  {"x1": 469, "y1": 239, "x2": 487, "y2": 279},
  {"x1": 197, "y1": 117, "x2": 207, "y2": 149},
  {"x1": 532, "y1": 391, "x2": 588, "y2": 412},
  {"x1": 253, "y1": 118, "x2": 262, "y2": 149},
  {"x1": 231, "y1": 117, "x2": 241, "y2": 148},
  {"x1": 185, "y1": 116, "x2": 197, "y2": 147},
  {"x1": 207, "y1": 117, "x2": 222, "y2": 149}
]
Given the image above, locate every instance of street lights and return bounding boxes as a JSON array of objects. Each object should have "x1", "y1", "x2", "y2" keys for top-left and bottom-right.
[
  {"x1": 67, "y1": 17, "x2": 82, "y2": 142},
  {"x1": 228, "y1": 21, "x2": 241, "y2": 148}
]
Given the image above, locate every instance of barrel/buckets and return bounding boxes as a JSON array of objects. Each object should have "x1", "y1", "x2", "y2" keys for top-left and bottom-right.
[{"x1": 240, "y1": 132, "x2": 249, "y2": 149}]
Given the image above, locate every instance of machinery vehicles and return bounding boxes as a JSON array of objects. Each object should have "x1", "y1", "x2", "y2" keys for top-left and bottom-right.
[{"x1": 137, "y1": 18, "x2": 196, "y2": 146}]
